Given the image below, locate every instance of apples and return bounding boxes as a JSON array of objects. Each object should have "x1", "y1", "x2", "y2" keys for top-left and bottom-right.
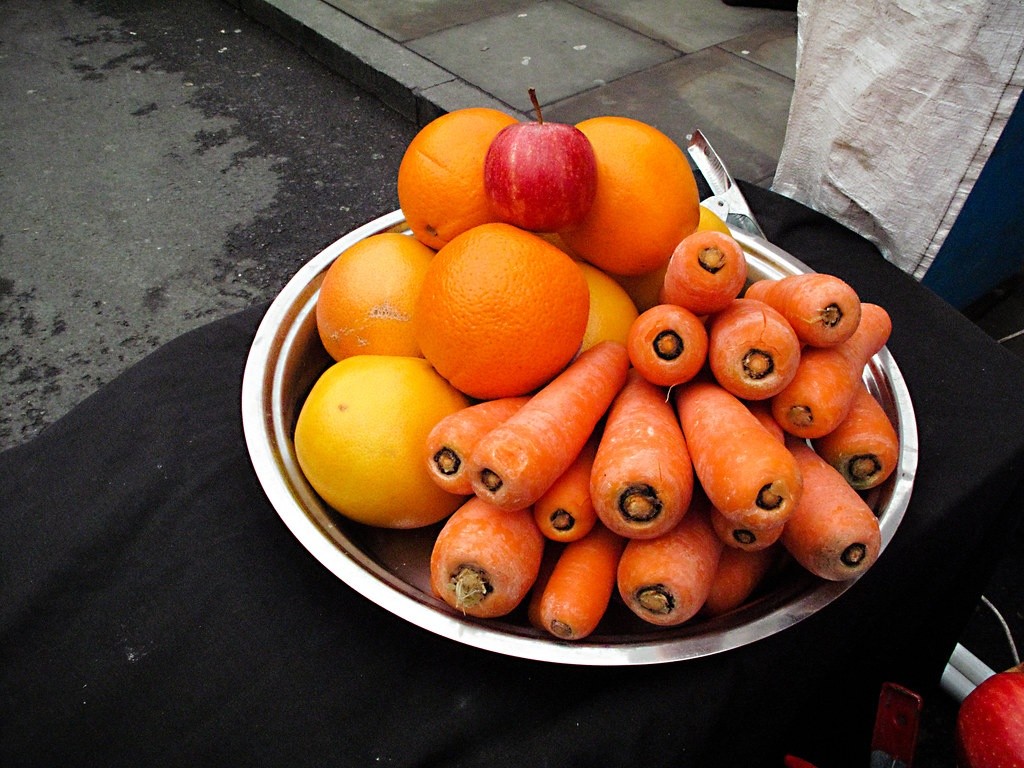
[
  {"x1": 483, "y1": 86, "x2": 599, "y2": 230},
  {"x1": 958, "y1": 660, "x2": 1024, "y2": 768}
]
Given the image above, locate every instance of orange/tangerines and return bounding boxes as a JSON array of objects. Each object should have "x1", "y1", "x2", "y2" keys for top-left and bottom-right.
[{"x1": 317, "y1": 105, "x2": 733, "y2": 397}]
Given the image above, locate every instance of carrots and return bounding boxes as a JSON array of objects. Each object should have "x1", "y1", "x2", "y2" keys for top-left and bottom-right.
[{"x1": 421, "y1": 230, "x2": 898, "y2": 640}]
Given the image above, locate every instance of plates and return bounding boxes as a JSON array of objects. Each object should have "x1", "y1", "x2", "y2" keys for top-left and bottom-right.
[{"x1": 240, "y1": 210, "x2": 919, "y2": 664}]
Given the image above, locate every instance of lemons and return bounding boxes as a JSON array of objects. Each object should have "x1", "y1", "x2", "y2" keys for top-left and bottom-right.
[{"x1": 292, "y1": 355, "x2": 468, "y2": 527}]
